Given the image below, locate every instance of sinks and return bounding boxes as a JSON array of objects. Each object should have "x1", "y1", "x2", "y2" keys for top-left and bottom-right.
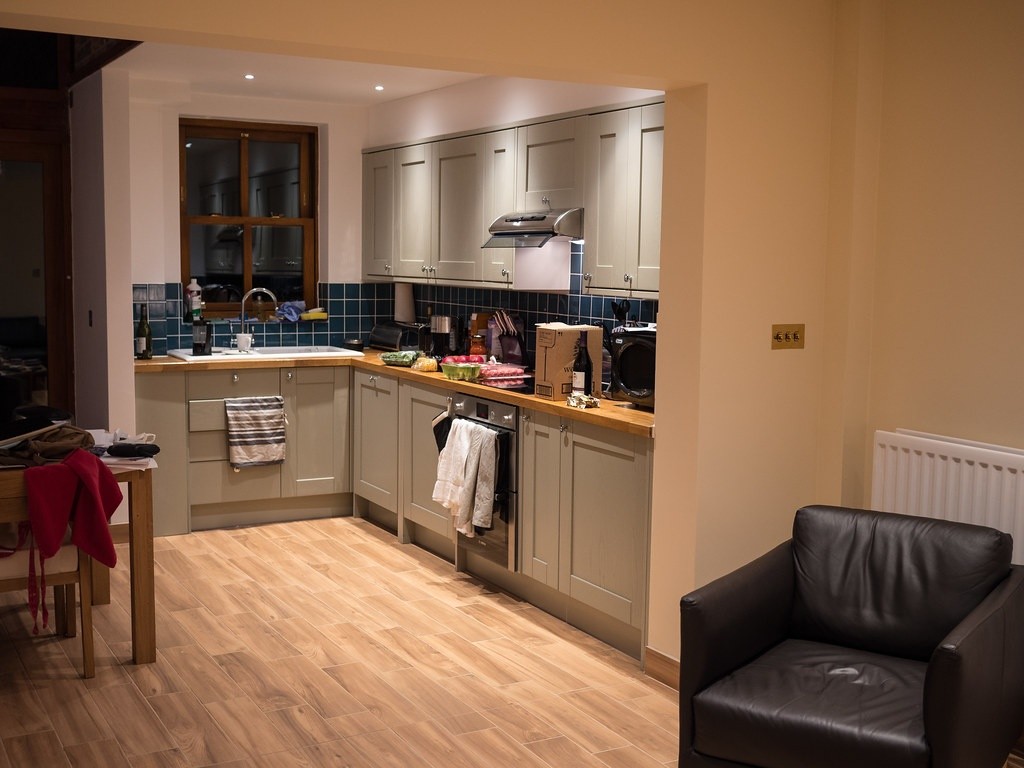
[{"x1": 254, "y1": 345, "x2": 365, "y2": 358}]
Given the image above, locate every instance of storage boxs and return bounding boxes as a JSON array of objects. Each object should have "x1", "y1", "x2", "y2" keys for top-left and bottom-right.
[{"x1": 534, "y1": 323, "x2": 604, "y2": 400}]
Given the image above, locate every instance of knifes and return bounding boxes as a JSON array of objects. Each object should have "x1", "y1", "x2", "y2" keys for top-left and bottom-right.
[{"x1": 491, "y1": 310, "x2": 518, "y2": 337}]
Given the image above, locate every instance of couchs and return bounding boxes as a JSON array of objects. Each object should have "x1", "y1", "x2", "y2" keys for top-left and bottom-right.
[{"x1": 676, "y1": 505, "x2": 1024, "y2": 768}]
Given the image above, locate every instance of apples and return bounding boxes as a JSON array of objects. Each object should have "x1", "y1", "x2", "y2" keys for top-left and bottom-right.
[{"x1": 440, "y1": 355, "x2": 484, "y2": 364}]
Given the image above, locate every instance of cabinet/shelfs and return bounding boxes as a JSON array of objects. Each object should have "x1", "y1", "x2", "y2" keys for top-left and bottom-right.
[
  {"x1": 361, "y1": 92, "x2": 665, "y2": 300},
  {"x1": 135, "y1": 365, "x2": 655, "y2": 662}
]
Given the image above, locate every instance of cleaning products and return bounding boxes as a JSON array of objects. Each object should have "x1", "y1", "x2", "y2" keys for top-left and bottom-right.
[{"x1": 185, "y1": 279, "x2": 201, "y2": 321}]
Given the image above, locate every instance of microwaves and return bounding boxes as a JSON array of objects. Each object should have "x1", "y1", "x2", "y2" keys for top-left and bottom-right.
[{"x1": 611, "y1": 333, "x2": 657, "y2": 411}]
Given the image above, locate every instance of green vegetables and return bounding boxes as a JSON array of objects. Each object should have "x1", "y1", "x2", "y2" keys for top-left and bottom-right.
[{"x1": 380, "y1": 350, "x2": 422, "y2": 367}]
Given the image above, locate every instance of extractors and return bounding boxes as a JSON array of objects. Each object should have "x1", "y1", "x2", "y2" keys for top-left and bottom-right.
[{"x1": 482, "y1": 207, "x2": 584, "y2": 248}]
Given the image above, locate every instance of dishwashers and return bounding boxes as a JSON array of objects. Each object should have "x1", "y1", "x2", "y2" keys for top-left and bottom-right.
[{"x1": 454, "y1": 392, "x2": 519, "y2": 573}]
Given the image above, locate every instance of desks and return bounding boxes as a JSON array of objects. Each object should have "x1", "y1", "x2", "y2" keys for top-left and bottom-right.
[{"x1": 99, "y1": 453, "x2": 157, "y2": 663}]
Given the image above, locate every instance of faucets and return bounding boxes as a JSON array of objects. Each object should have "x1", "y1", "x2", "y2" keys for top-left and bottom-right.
[{"x1": 241, "y1": 287, "x2": 277, "y2": 333}]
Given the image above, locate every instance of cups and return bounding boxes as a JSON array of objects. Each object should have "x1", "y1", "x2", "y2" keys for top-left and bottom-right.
[
  {"x1": 192, "y1": 316, "x2": 212, "y2": 355},
  {"x1": 235, "y1": 334, "x2": 252, "y2": 352}
]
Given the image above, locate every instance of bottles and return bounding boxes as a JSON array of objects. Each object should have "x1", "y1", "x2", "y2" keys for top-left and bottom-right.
[
  {"x1": 469, "y1": 335, "x2": 488, "y2": 362},
  {"x1": 187, "y1": 279, "x2": 203, "y2": 320},
  {"x1": 184, "y1": 289, "x2": 193, "y2": 322},
  {"x1": 571, "y1": 330, "x2": 593, "y2": 397},
  {"x1": 136, "y1": 303, "x2": 152, "y2": 359},
  {"x1": 252, "y1": 296, "x2": 266, "y2": 320}
]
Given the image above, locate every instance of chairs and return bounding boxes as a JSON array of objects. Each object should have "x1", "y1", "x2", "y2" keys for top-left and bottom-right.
[{"x1": 0, "y1": 363, "x2": 112, "y2": 679}]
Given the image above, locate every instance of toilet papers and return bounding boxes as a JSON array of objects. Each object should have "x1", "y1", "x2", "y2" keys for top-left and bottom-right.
[{"x1": 394, "y1": 282, "x2": 416, "y2": 322}]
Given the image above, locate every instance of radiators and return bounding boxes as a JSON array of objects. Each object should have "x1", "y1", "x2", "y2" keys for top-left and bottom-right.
[{"x1": 870, "y1": 428, "x2": 1024, "y2": 565}]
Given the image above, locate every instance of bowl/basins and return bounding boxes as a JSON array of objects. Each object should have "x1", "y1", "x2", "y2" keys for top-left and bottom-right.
[
  {"x1": 440, "y1": 362, "x2": 482, "y2": 380},
  {"x1": 344, "y1": 339, "x2": 364, "y2": 352}
]
